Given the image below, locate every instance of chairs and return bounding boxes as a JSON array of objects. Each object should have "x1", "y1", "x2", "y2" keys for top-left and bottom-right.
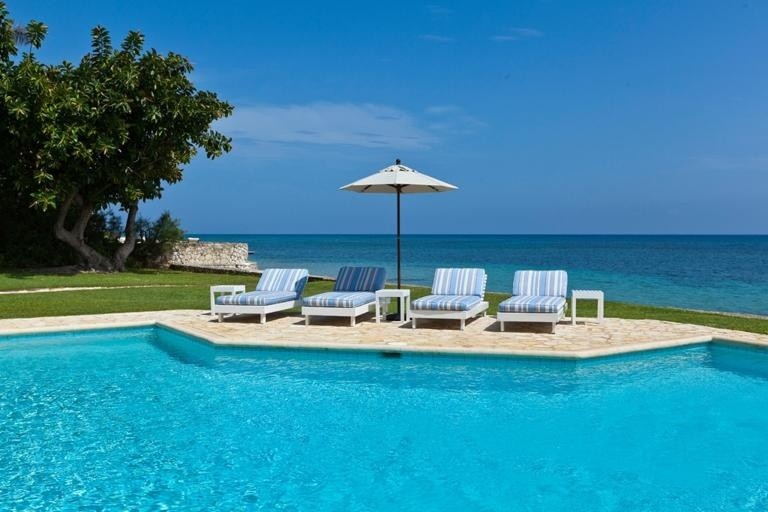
[
  {"x1": 211, "y1": 268, "x2": 309, "y2": 323},
  {"x1": 496, "y1": 270, "x2": 568, "y2": 334},
  {"x1": 407, "y1": 268, "x2": 489, "y2": 332},
  {"x1": 301, "y1": 266, "x2": 390, "y2": 326}
]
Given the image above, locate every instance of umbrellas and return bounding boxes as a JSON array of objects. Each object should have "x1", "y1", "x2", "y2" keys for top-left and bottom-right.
[{"x1": 338, "y1": 157, "x2": 461, "y2": 315}]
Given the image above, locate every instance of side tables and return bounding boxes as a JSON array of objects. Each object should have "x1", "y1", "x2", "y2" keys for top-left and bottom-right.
[
  {"x1": 210, "y1": 284, "x2": 246, "y2": 317},
  {"x1": 571, "y1": 290, "x2": 604, "y2": 326},
  {"x1": 375, "y1": 288, "x2": 411, "y2": 325}
]
